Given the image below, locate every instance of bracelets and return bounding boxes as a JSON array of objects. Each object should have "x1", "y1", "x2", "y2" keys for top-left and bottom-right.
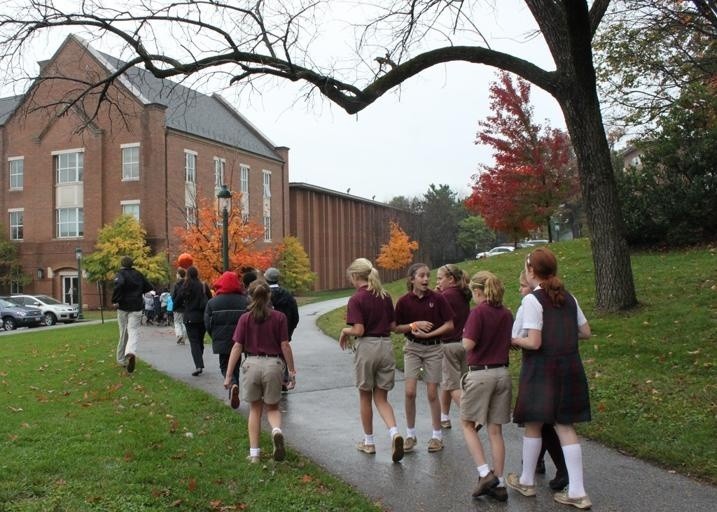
[
  {"x1": 409, "y1": 322, "x2": 417, "y2": 331},
  {"x1": 288, "y1": 369, "x2": 296, "y2": 378}
]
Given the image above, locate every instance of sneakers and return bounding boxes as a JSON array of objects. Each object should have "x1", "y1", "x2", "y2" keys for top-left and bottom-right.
[
  {"x1": 281, "y1": 379, "x2": 289, "y2": 394},
  {"x1": 441, "y1": 419, "x2": 451, "y2": 429},
  {"x1": 141, "y1": 318, "x2": 173, "y2": 327},
  {"x1": 505, "y1": 472, "x2": 536, "y2": 497},
  {"x1": 487, "y1": 486, "x2": 508, "y2": 502},
  {"x1": 474, "y1": 421, "x2": 483, "y2": 432},
  {"x1": 356, "y1": 440, "x2": 377, "y2": 455},
  {"x1": 176, "y1": 335, "x2": 186, "y2": 345},
  {"x1": 403, "y1": 434, "x2": 418, "y2": 453},
  {"x1": 270, "y1": 428, "x2": 286, "y2": 462},
  {"x1": 549, "y1": 470, "x2": 569, "y2": 490},
  {"x1": 245, "y1": 456, "x2": 261, "y2": 466},
  {"x1": 428, "y1": 437, "x2": 445, "y2": 453},
  {"x1": 521, "y1": 457, "x2": 546, "y2": 474},
  {"x1": 192, "y1": 367, "x2": 203, "y2": 377},
  {"x1": 471, "y1": 469, "x2": 500, "y2": 497},
  {"x1": 553, "y1": 489, "x2": 593, "y2": 509},
  {"x1": 228, "y1": 385, "x2": 241, "y2": 409},
  {"x1": 391, "y1": 434, "x2": 404, "y2": 462},
  {"x1": 122, "y1": 351, "x2": 137, "y2": 375}
]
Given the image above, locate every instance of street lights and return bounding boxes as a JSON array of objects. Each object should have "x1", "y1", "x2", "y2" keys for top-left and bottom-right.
[
  {"x1": 216, "y1": 184, "x2": 231, "y2": 272},
  {"x1": 74, "y1": 247, "x2": 85, "y2": 320}
]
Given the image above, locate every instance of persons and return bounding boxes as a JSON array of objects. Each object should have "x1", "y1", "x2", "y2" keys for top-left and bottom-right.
[
  {"x1": 173, "y1": 269, "x2": 185, "y2": 343},
  {"x1": 112, "y1": 257, "x2": 153, "y2": 373},
  {"x1": 204, "y1": 270, "x2": 251, "y2": 409},
  {"x1": 512, "y1": 247, "x2": 591, "y2": 508},
  {"x1": 244, "y1": 272, "x2": 256, "y2": 291},
  {"x1": 224, "y1": 279, "x2": 295, "y2": 466},
  {"x1": 433, "y1": 263, "x2": 483, "y2": 431},
  {"x1": 339, "y1": 258, "x2": 404, "y2": 461},
  {"x1": 459, "y1": 270, "x2": 513, "y2": 502},
  {"x1": 173, "y1": 266, "x2": 212, "y2": 376},
  {"x1": 394, "y1": 262, "x2": 454, "y2": 452},
  {"x1": 263, "y1": 268, "x2": 299, "y2": 394},
  {"x1": 142, "y1": 290, "x2": 173, "y2": 327},
  {"x1": 517, "y1": 269, "x2": 568, "y2": 490}
]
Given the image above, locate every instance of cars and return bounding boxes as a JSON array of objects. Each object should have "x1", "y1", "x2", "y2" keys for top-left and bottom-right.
[
  {"x1": 0, "y1": 292, "x2": 77, "y2": 331},
  {"x1": 474, "y1": 238, "x2": 549, "y2": 260}
]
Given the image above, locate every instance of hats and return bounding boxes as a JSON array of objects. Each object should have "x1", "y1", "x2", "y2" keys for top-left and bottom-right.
[{"x1": 263, "y1": 267, "x2": 281, "y2": 282}]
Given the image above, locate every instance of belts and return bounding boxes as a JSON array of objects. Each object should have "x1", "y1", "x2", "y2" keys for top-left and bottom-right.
[
  {"x1": 442, "y1": 337, "x2": 463, "y2": 345},
  {"x1": 358, "y1": 331, "x2": 391, "y2": 338},
  {"x1": 405, "y1": 334, "x2": 441, "y2": 346},
  {"x1": 467, "y1": 362, "x2": 510, "y2": 371},
  {"x1": 245, "y1": 352, "x2": 282, "y2": 359}
]
